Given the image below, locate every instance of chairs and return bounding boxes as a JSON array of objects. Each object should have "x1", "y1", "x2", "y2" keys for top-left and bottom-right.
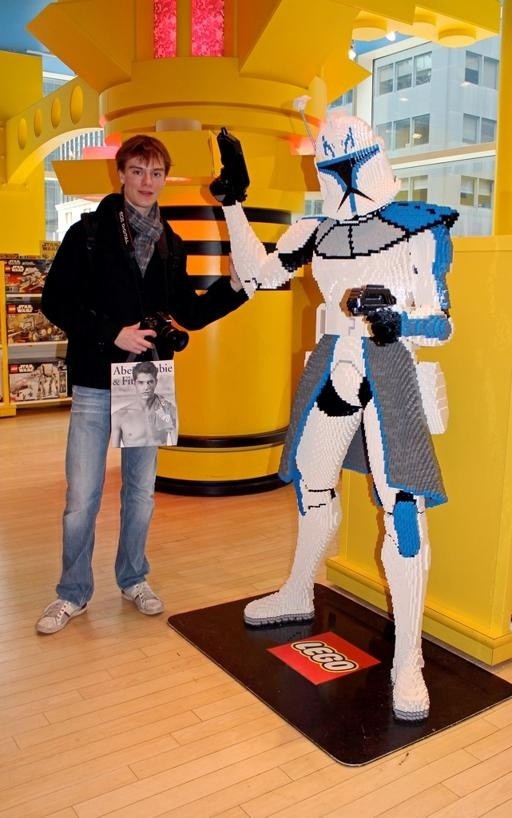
[
  {"x1": 122, "y1": 581, "x2": 163, "y2": 615},
  {"x1": 36, "y1": 597, "x2": 87, "y2": 634}
]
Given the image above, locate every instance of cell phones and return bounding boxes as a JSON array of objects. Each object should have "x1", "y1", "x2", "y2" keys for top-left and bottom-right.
[{"x1": 138, "y1": 313, "x2": 189, "y2": 353}]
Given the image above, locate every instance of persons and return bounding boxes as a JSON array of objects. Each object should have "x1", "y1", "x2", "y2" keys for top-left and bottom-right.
[
  {"x1": 111, "y1": 360, "x2": 176, "y2": 448},
  {"x1": 36, "y1": 132, "x2": 249, "y2": 633}
]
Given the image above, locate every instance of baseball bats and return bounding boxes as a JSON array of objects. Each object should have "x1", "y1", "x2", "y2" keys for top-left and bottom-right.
[
  {"x1": 347, "y1": 284, "x2": 397, "y2": 346},
  {"x1": 210, "y1": 127, "x2": 249, "y2": 204}
]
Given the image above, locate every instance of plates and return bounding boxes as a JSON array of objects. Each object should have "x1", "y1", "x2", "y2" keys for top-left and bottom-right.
[{"x1": 0, "y1": 253, "x2": 72, "y2": 404}]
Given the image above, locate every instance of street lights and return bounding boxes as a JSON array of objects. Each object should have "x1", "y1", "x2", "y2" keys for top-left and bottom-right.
[{"x1": 111, "y1": 344, "x2": 179, "y2": 448}]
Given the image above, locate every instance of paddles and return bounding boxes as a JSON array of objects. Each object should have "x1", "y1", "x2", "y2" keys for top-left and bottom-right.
[{"x1": 168, "y1": 583, "x2": 511, "y2": 767}]
